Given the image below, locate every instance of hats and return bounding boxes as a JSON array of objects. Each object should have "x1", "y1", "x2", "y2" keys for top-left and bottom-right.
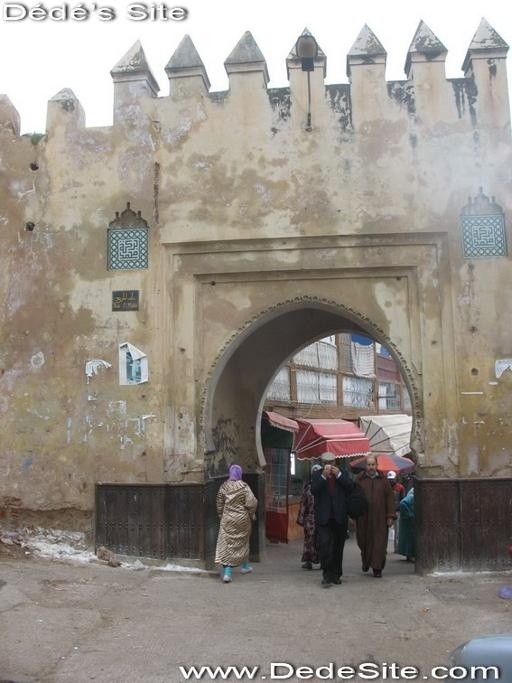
[
  {"x1": 321, "y1": 452, "x2": 335, "y2": 461},
  {"x1": 387, "y1": 470, "x2": 396, "y2": 480}
]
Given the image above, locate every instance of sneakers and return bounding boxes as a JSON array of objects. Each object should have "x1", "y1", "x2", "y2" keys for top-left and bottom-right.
[
  {"x1": 321, "y1": 578, "x2": 331, "y2": 587},
  {"x1": 301, "y1": 562, "x2": 312, "y2": 569},
  {"x1": 239, "y1": 566, "x2": 254, "y2": 574},
  {"x1": 362, "y1": 565, "x2": 369, "y2": 571},
  {"x1": 223, "y1": 575, "x2": 232, "y2": 582},
  {"x1": 334, "y1": 578, "x2": 342, "y2": 584},
  {"x1": 373, "y1": 568, "x2": 383, "y2": 577}
]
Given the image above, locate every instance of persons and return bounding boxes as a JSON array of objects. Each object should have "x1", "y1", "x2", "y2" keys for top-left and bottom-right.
[
  {"x1": 214, "y1": 464, "x2": 259, "y2": 583},
  {"x1": 297, "y1": 451, "x2": 414, "y2": 585}
]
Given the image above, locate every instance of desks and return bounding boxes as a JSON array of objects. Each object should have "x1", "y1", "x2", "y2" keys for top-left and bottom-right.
[{"x1": 264, "y1": 501, "x2": 306, "y2": 544}]
